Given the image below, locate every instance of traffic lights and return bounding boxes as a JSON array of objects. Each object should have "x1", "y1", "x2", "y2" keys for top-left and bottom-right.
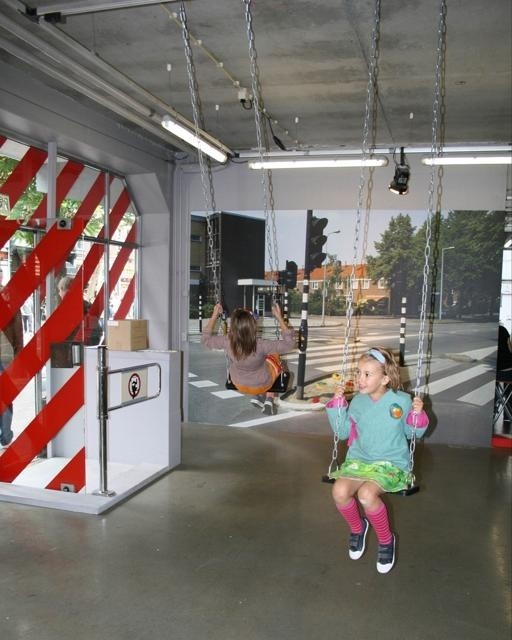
[
  {"x1": 310, "y1": 217, "x2": 327, "y2": 267},
  {"x1": 285, "y1": 261, "x2": 296, "y2": 288}
]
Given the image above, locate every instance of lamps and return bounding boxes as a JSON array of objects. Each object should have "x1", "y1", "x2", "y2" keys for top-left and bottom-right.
[
  {"x1": 422, "y1": 156, "x2": 512, "y2": 166},
  {"x1": 161, "y1": 115, "x2": 227, "y2": 164},
  {"x1": 389, "y1": 148, "x2": 410, "y2": 196},
  {"x1": 248, "y1": 156, "x2": 389, "y2": 170}
]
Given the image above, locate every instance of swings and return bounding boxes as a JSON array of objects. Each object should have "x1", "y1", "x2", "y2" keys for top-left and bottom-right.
[
  {"x1": 178, "y1": 0, "x2": 290, "y2": 393},
  {"x1": 321, "y1": 1, "x2": 447, "y2": 498}
]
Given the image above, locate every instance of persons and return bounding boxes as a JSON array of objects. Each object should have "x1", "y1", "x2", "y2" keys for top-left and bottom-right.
[
  {"x1": 496, "y1": 326, "x2": 511, "y2": 436},
  {"x1": 20, "y1": 296, "x2": 34, "y2": 334},
  {"x1": 58, "y1": 278, "x2": 103, "y2": 347},
  {"x1": 325, "y1": 345, "x2": 431, "y2": 574},
  {"x1": 0, "y1": 270, "x2": 26, "y2": 451},
  {"x1": 197, "y1": 301, "x2": 298, "y2": 417}
]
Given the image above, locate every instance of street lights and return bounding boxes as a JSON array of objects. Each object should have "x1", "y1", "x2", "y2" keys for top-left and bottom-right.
[
  {"x1": 439, "y1": 246, "x2": 456, "y2": 320},
  {"x1": 321, "y1": 230, "x2": 340, "y2": 326}
]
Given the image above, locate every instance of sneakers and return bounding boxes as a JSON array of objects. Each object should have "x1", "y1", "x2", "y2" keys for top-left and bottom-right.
[
  {"x1": 249, "y1": 396, "x2": 264, "y2": 408},
  {"x1": 259, "y1": 396, "x2": 276, "y2": 416},
  {"x1": 375, "y1": 530, "x2": 396, "y2": 575},
  {"x1": 347, "y1": 516, "x2": 370, "y2": 562}
]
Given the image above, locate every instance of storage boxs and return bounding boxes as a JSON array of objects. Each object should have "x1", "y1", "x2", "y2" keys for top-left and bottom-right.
[{"x1": 105, "y1": 319, "x2": 147, "y2": 351}]
